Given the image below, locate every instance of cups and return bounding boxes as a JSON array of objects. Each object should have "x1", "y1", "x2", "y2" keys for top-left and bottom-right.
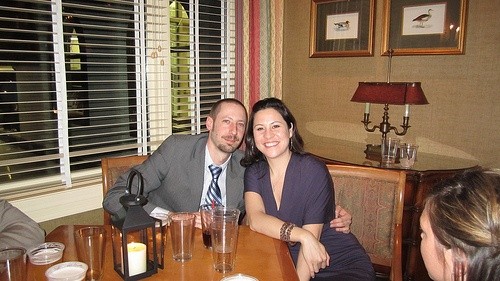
[
  {"x1": 73, "y1": 226, "x2": 107, "y2": 281},
  {"x1": 0, "y1": 246, "x2": 27, "y2": 281},
  {"x1": 199, "y1": 204, "x2": 224, "y2": 250},
  {"x1": 169, "y1": 211, "x2": 197, "y2": 263},
  {"x1": 44, "y1": 261, "x2": 89, "y2": 281},
  {"x1": 400, "y1": 142, "x2": 419, "y2": 168},
  {"x1": 26, "y1": 241, "x2": 66, "y2": 281},
  {"x1": 148, "y1": 211, "x2": 169, "y2": 255},
  {"x1": 220, "y1": 274, "x2": 260, "y2": 281},
  {"x1": 379, "y1": 137, "x2": 400, "y2": 164},
  {"x1": 210, "y1": 207, "x2": 240, "y2": 273}
]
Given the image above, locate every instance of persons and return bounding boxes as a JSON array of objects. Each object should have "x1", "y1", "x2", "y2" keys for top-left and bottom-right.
[
  {"x1": 0, "y1": 199, "x2": 45, "y2": 260},
  {"x1": 103, "y1": 99, "x2": 247, "y2": 230},
  {"x1": 420, "y1": 171, "x2": 500, "y2": 281},
  {"x1": 241, "y1": 98, "x2": 376, "y2": 281}
]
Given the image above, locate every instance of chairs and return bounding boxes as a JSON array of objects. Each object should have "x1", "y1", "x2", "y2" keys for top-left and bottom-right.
[
  {"x1": 322, "y1": 162, "x2": 406, "y2": 280},
  {"x1": 101, "y1": 154, "x2": 148, "y2": 225}
]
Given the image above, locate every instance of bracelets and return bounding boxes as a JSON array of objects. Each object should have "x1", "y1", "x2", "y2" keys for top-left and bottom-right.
[{"x1": 280, "y1": 221, "x2": 297, "y2": 246}]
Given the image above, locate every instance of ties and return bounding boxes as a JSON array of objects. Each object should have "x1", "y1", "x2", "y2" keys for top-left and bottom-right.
[{"x1": 203, "y1": 164, "x2": 222, "y2": 210}]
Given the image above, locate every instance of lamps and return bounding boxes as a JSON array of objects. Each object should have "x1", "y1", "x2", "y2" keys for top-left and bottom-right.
[{"x1": 348, "y1": 81, "x2": 430, "y2": 160}]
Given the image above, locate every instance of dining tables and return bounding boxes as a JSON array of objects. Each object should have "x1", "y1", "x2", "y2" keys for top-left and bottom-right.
[{"x1": 0, "y1": 222, "x2": 304, "y2": 281}]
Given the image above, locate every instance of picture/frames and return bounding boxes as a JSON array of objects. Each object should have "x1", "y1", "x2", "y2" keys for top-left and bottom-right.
[
  {"x1": 380, "y1": 1, "x2": 467, "y2": 56},
  {"x1": 308, "y1": 1, "x2": 377, "y2": 59}
]
